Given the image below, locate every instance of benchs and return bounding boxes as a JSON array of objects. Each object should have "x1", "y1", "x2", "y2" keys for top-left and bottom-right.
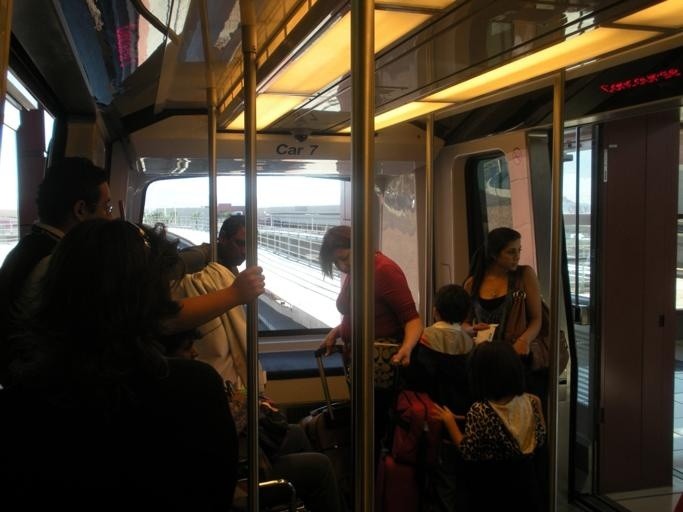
[{"x1": 258, "y1": 349, "x2": 349, "y2": 403}]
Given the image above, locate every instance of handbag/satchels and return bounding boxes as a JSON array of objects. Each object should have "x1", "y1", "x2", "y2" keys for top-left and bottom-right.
[{"x1": 501, "y1": 265, "x2": 571, "y2": 381}]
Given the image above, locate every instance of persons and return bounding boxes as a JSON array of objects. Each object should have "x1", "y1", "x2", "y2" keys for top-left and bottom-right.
[
  {"x1": 1, "y1": 159, "x2": 339, "y2": 511},
  {"x1": 319, "y1": 225, "x2": 547, "y2": 511}
]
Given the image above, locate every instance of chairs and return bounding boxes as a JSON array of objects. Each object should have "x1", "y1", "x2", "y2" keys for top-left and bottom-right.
[{"x1": 254, "y1": 478, "x2": 304, "y2": 511}]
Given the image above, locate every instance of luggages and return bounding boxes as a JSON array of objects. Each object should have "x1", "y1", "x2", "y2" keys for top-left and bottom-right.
[
  {"x1": 295, "y1": 342, "x2": 381, "y2": 512},
  {"x1": 382, "y1": 388, "x2": 444, "y2": 511}
]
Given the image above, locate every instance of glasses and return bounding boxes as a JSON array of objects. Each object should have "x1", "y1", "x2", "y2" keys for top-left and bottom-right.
[{"x1": 84, "y1": 198, "x2": 120, "y2": 218}]
